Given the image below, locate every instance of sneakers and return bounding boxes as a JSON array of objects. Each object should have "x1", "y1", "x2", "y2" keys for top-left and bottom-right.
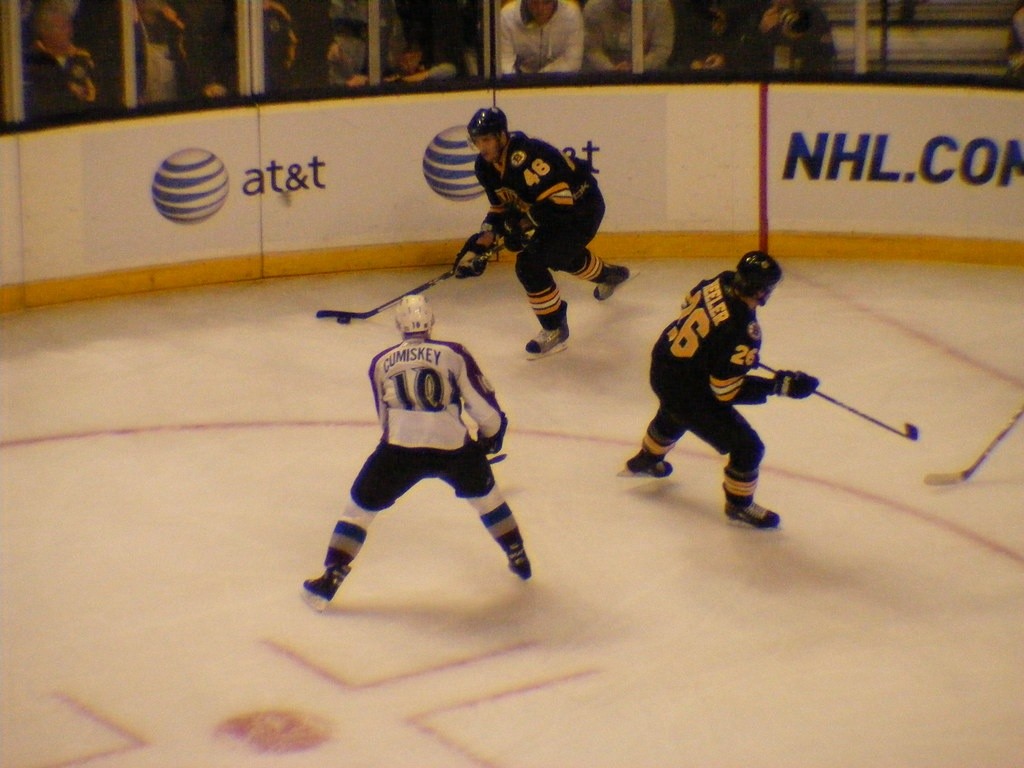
[
  {"x1": 525, "y1": 312, "x2": 570, "y2": 361},
  {"x1": 508, "y1": 540, "x2": 532, "y2": 580},
  {"x1": 593, "y1": 264, "x2": 631, "y2": 302},
  {"x1": 299, "y1": 565, "x2": 354, "y2": 611},
  {"x1": 724, "y1": 500, "x2": 781, "y2": 531},
  {"x1": 626, "y1": 449, "x2": 673, "y2": 478}
]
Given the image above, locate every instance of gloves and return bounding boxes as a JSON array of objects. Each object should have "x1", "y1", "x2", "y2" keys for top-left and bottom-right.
[
  {"x1": 453, "y1": 244, "x2": 492, "y2": 279},
  {"x1": 771, "y1": 369, "x2": 819, "y2": 400},
  {"x1": 503, "y1": 214, "x2": 530, "y2": 252}
]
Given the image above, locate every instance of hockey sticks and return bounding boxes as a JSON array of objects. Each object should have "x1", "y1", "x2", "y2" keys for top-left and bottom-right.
[
  {"x1": 313, "y1": 223, "x2": 521, "y2": 324},
  {"x1": 755, "y1": 359, "x2": 918, "y2": 440},
  {"x1": 921, "y1": 404, "x2": 1024, "y2": 486}
]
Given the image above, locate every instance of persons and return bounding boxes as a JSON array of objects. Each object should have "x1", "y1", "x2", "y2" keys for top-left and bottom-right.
[
  {"x1": 627, "y1": 250, "x2": 819, "y2": 529},
  {"x1": 21, "y1": 0, "x2": 839, "y2": 122},
  {"x1": 456, "y1": 105, "x2": 628, "y2": 353},
  {"x1": 304, "y1": 296, "x2": 532, "y2": 602},
  {"x1": 1001, "y1": 0, "x2": 1024, "y2": 88}
]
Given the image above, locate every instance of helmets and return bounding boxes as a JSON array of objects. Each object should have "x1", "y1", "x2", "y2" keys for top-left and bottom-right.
[
  {"x1": 467, "y1": 107, "x2": 508, "y2": 148},
  {"x1": 393, "y1": 294, "x2": 435, "y2": 338},
  {"x1": 734, "y1": 250, "x2": 783, "y2": 307}
]
